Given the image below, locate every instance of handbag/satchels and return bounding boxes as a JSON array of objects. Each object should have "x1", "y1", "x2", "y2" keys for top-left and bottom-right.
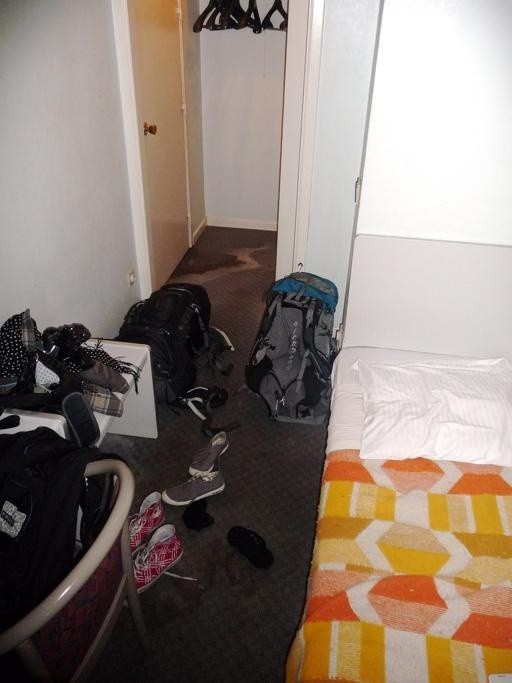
[{"x1": 116, "y1": 282, "x2": 211, "y2": 402}]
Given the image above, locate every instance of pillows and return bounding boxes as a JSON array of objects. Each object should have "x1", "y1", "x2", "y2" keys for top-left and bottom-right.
[{"x1": 354, "y1": 358, "x2": 510, "y2": 467}]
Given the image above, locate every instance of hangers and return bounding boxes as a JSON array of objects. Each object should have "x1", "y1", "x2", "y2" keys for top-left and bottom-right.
[{"x1": 192, "y1": 0, "x2": 288, "y2": 33}]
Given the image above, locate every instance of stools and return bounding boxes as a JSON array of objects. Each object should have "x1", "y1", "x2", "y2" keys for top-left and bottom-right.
[{"x1": 80, "y1": 336, "x2": 160, "y2": 448}]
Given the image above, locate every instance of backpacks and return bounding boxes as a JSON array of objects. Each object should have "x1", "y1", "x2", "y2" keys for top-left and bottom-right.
[{"x1": 245, "y1": 271, "x2": 340, "y2": 427}]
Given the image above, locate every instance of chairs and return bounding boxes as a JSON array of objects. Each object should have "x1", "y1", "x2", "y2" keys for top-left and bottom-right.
[{"x1": 0, "y1": 458, "x2": 153, "y2": 683}]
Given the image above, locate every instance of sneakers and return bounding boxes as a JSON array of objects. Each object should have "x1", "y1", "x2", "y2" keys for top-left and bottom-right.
[
  {"x1": 127, "y1": 491, "x2": 165, "y2": 551},
  {"x1": 187, "y1": 431, "x2": 230, "y2": 476},
  {"x1": 133, "y1": 523, "x2": 184, "y2": 592},
  {"x1": 162, "y1": 471, "x2": 225, "y2": 507}
]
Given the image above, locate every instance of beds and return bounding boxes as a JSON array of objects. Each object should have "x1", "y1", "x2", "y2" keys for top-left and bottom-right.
[{"x1": 284, "y1": 233, "x2": 512, "y2": 683}]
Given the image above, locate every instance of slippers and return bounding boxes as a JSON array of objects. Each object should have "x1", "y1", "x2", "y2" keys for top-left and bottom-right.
[{"x1": 227, "y1": 525, "x2": 274, "y2": 568}]
